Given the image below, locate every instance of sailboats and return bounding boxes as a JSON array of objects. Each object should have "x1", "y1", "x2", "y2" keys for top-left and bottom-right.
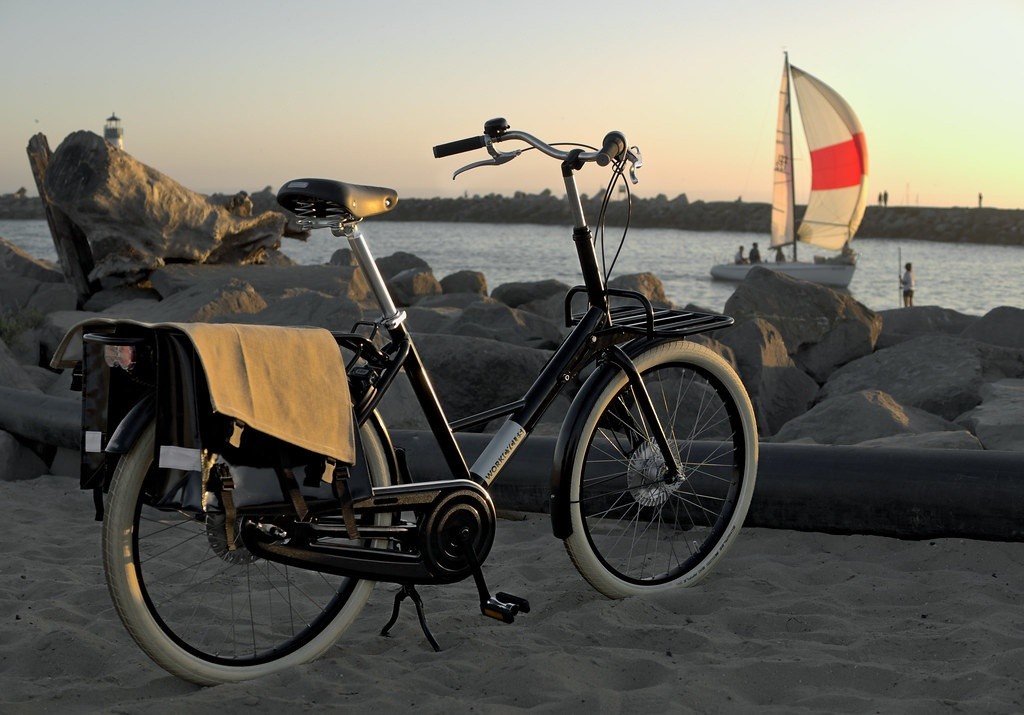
[{"x1": 709, "y1": 51, "x2": 869, "y2": 288}]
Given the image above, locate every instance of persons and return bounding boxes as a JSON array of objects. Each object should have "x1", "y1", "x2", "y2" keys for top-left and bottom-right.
[
  {"x1": 883, "y1": 190, "x2": 888, "y2": 207},
  {"x1": 749, "y1": 242, "x2": 760, "y2": 264},
  {"x1": 979, "y1": 193, "x2": 983, "y2": 207},
  {"x1": 842, "y1": 240, "x2": 853, "y2": 256},
  {"x1": 735, "y1": 246, "x2": 747, "y2": 264},
  {"x1": 899, "y1": 264, "x2": 915, "y2": 307},
  {"x1": 879, "y1": 193, "x2": 882, "y2": 205},
  {"x1": 776, "y1": 247, "x2": 784, "y2": 262}
]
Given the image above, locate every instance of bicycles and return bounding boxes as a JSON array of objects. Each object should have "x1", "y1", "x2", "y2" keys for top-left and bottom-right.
[{"x1": 69, "y1": 117, "x2": 762, "y2": 687}]
